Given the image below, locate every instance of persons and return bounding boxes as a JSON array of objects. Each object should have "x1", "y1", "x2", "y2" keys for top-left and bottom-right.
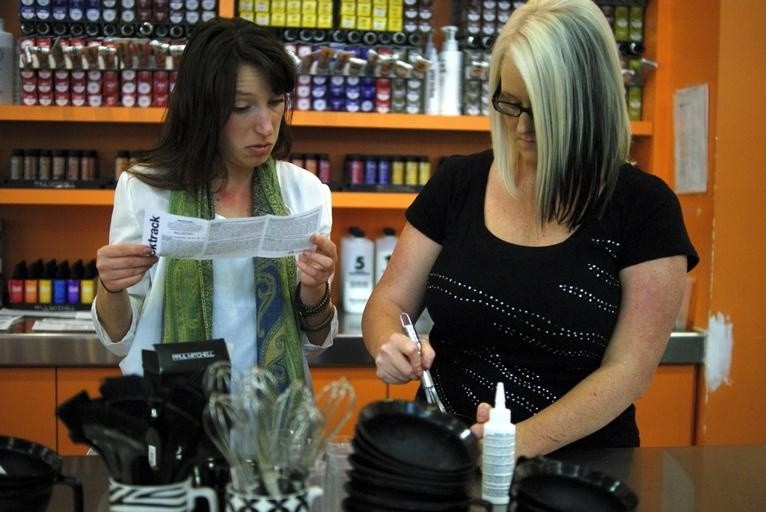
[
  {"x1": 88, "y1": 16, "x2": 339, "y2": 408},
  {"x1": 362, "y1": 1, "x2": 701, "y2": 471}
]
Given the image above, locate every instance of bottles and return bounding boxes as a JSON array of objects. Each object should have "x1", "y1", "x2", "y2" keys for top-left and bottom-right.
[{"x1": 478, "y1": 379, "x2": 523, "y2": 506}]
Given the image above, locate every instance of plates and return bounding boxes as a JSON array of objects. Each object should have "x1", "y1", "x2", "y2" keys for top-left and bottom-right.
[
  {"x1": 506, "y1": 454, "x2": 639, "y2": 512},
  {"x1": 344, "y1": 400, "x2": 482, "y2": 512}
]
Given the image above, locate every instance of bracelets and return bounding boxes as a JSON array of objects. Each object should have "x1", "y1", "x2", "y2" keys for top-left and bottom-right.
[
  {"x1": 293, "y1": 280, "x2": 332, "y2": 316},
  {"x1": 298, "y1": 307, "x2": 336, "y2": 331},
  {"x1": 98, "y1": 273, "x2": 124, "y2": 294}
]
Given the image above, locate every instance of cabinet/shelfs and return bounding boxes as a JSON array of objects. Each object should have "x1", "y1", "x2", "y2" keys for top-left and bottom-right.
[{"x1": 0, "y1": 0, "x2": 722, "y2": 443}]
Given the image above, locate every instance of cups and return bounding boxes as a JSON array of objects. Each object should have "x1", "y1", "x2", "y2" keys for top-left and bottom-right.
[
  {"x1": 225, "y1": 478, "x2": 326, "y2": 511},
  {"x1": 107, "y1": 475, "x2": 218, "y2": 512}
]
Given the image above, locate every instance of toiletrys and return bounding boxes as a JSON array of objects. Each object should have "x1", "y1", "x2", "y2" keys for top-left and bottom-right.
[
  {"x1": 2, "y1": 0, "x2": 654, "y2": 129},
  {"x1": 0, "y1": 143, "x2": 434, "y2": 200},
  {"x1": 0, "y1": 255, "x2": 101, "y2": 313},
  {"x1": 337, "y1": 223, "x2": 400, "y2": 317}
]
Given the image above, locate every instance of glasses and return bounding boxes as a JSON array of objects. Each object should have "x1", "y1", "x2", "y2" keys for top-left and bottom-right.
[{"x1": 490, "y1": 76, "x2": 533, "y2": 121}]
